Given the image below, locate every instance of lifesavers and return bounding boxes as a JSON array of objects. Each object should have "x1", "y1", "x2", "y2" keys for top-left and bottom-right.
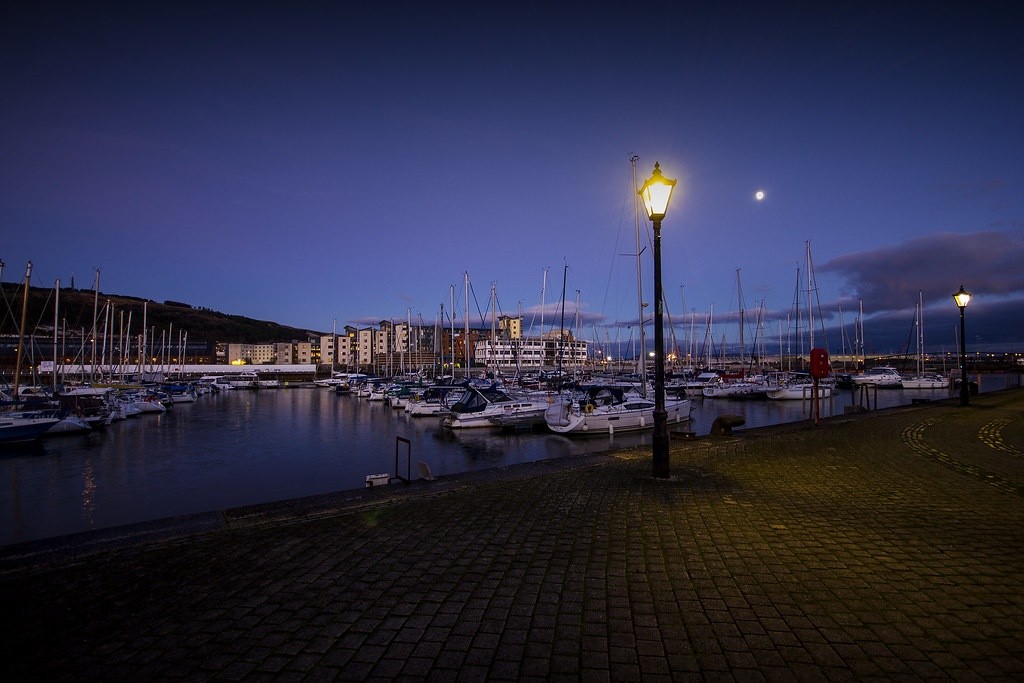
[
  {"x1": 585, "y1": 404, "x2": 593, "y2": 412},
  {"x1": 415, "y1": 395, "x2": 419, "y2": 400}
]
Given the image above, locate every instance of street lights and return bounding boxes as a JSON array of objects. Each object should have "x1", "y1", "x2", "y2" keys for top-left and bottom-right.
[
  {"x1": 637, "y1": 159, "x2": 679, "y2": 479},
  {"x1": 952, "y1": 285, "x2": 972, "y2": 406}
]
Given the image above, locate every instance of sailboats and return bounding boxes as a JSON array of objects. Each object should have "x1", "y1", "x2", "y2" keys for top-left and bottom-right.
[{"x1": 0, "y1": 261, "x2": 259, "y2": 448}]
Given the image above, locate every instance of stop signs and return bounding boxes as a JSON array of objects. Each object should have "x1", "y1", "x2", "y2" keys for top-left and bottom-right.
[{"x1": 312, "y1": 236, "x2": 966, "y2": 433}]
